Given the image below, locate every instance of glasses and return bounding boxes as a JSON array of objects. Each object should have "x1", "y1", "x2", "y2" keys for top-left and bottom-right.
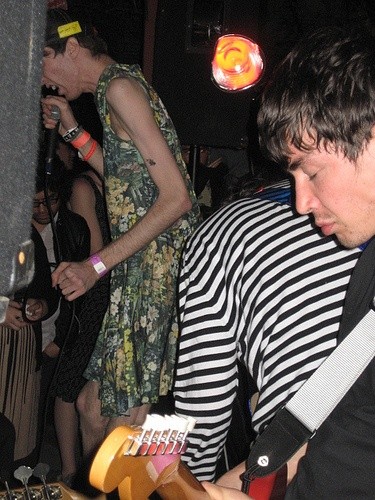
[{"x1": 33, "y1": 193, "x2": 59, "y2": 209}]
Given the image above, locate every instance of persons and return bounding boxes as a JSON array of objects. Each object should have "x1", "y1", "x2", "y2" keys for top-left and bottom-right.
[
  {"x1": 43, "y1": 5, "x2": 207, "y2": 500},
  {"x1": 0, "y1": 217, "x2": 59, "y2": 490},
  {"x1": 176, "y1": 131, "x2": 298, "y2": 230},
  {"x1": 17, "y1": 84, "x2": 119, "y2": 495},
  {"x1": 197, "y1": 22, "x2": 375, "y2": 499},
  {"x1": 173, "y1": 167, "x2": 367, "y2": 485}
]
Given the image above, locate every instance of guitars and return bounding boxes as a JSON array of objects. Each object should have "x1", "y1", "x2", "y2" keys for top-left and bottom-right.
[
  {"x1": 0, "y1": 463, "x2": 89, "y2": 500},
  {"x1": 89, "y1": 413, "x2": 288, "y2": 500}
]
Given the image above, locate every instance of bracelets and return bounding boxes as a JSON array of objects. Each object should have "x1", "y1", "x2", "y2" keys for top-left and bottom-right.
[
  {"x1": 70, "y1": 129, "x2": 91, "y2": 149},
  {"x1": 88, "y1": 253, "x2": 108, "y2": 278},
  {"x1": 62, "y1": 124, "x2": 85, "y2": 144},
  {"x1": 78, "y1": 140, "x2": 97, "y2": 162}
]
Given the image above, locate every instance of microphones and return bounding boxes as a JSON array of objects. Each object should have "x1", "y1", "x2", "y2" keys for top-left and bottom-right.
[{"x1": 45, "y1": 104, "x2": 61, "y2": 176}]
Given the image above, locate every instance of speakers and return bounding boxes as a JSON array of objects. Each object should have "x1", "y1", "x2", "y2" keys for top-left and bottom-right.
[{"x1": 151, "y1": 0, "x2": 278, "y2": 150}]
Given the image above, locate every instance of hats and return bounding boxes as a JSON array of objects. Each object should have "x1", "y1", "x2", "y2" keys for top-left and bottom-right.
[{"x1": 45, "y1": 7, "x2": 88, "y2": 44}]
